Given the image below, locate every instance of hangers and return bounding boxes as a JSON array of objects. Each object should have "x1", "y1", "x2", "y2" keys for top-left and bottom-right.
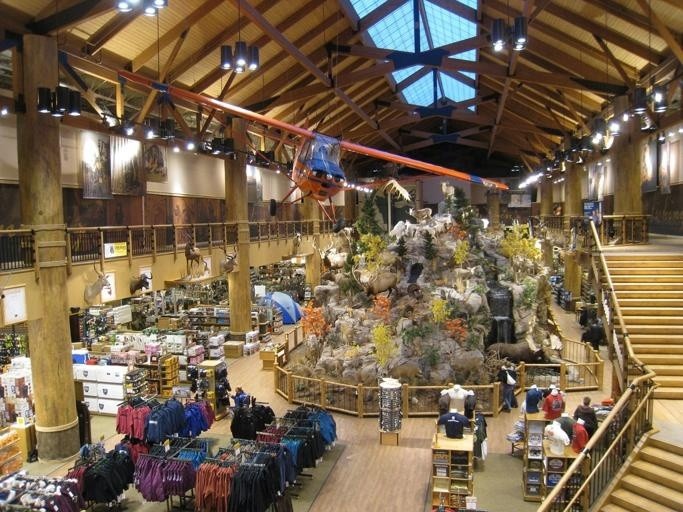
[{"x1": 0, "y1": 392, "x2": 329, "y2": 512}]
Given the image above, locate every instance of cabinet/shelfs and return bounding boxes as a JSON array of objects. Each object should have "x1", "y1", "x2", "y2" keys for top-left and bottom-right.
[
  {"x1": 426, "y1": 383, "x2": 623, "y2": 512},
  {"x1": 548, "y1": 245, "x2": 602, "y2": 329},
  {"x1": 0, "y1": 257, "x2": 306, "y2": 480},
  {"x1": 376, "y1": 379, "x2": 403, "y2": 444}
]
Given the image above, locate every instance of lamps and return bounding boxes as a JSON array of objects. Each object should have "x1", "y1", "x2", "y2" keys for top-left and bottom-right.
[
  {"x1": 490, "y1": 0, "x2": 527, "y2": 52},
  {"x1": 37, "y1": 0, "x2": 275, "y2": 170},
  {"x1": 540, "y1": 0, "x2": 667, "y2": 179}
]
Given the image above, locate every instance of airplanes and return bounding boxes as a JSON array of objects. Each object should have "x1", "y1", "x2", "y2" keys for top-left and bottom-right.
[{"x1": 118, "y1": 68, "x2": 510, "y2": 234}]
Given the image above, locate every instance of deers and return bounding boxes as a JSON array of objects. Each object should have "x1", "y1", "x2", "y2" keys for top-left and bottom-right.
[
  {"x1": 185, "y1": 233, "x2": 207, "y2": 273},
  {"x1": 405, "y1": 207, "x2": 432, "y2": 224},
  {"x1": 219, "y1": 244, "x2": 238, "y2": 274},
  {"x1": 312, "y1": 235, "x2": 349, "y2": 274},
  {"x1": 292, "y1": 232, "x2": 301, "y2": 255},
  {"x1": 352, "y1": 264, "x2": 400, "y2": 298},
  {"x1": 441, "y1": 181, "x2": 455, "y2": 201}
]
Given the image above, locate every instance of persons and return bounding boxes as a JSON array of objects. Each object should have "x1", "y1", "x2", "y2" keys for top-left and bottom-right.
[
  {"x1": 580, "y1": 319, "x2": 603, "y2": 351},
  {"x1": 496, "y1": 358, "x2": 519, "y2": 412},
  {"x1": 572, "y1": 397, "x2": 599, "y2": 439},
  {"x1": 435, "y1": 409, "x2": 471, "y2": 439},
  {"x1": 435, "y1": 384, "x2": 470, "y2": 416},
  {"x1": 228, "y1": 387, "x2": 246, "y2": 409},
  {"x1": 525, "y1": 384, "x2": 588, "y2": 454}
]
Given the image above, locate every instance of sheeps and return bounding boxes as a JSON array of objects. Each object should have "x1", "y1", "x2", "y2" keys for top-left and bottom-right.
[{"x1": 84, "y1": 258, "x2": 110, "y2": 305}]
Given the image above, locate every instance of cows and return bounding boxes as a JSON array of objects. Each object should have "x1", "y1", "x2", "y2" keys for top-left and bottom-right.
[{"x1": 130, "y1": 272, "x2": 152, "y2": 295}]
